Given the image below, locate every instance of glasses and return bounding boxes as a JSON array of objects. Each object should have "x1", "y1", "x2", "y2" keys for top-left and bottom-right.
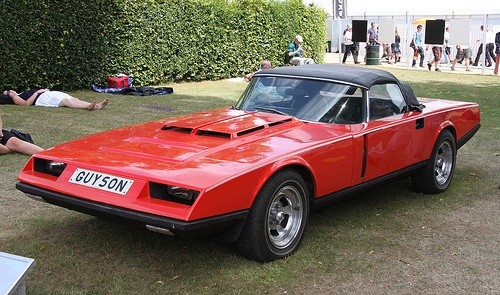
[{"x1": 7, "y1": 89, "x2": 10, "y2": 96}]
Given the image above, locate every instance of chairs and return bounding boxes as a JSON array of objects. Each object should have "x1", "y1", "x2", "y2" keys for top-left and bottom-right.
[{"x1": 290, "y1": 81, "x2": 328, "y2": 116}]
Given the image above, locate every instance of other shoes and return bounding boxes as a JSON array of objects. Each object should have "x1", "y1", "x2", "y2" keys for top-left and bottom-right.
[
  {"x1": 465, "y1": 61, "x2": 473, "y2": 65},
  {"x1": 412, "y1": 64, "x2": 417, "y2": 68},
  {"x1": 486, "y1": 64, "x2": 492, "y2": 67},
  {"x1": 397, "y1": 57, "x2": 400, "y2": 62},
  {"x1": 472, "y1": 63, "x2": 477, "y2": 66},
  {"x1": 435, "y1": 68, "x2": 441, "y2": 72},
  {"x1": 343, "y1": 61, "x2": 346, "y2": 64},
  {"x1": 355, "y1": 61, "x2": 361, "y2": 64},
  {"x1": 457, "y1": 61, "x2": 462, "y2": 64},
  {"x1": 385, "y1": 57, "x2": 392, "y2": 60},
  {"x1": 420, "y1": 65, "x2": 425, "y2": 68},
  {"x1": 466, "y1": 69, "x2": 470, "y2": 71},
  {"x1": 427, "y1": 63, "x2": 433, "y2": 71},
  {"x1": 451, "y1": 66, "x2": 455, "y2": 70}
]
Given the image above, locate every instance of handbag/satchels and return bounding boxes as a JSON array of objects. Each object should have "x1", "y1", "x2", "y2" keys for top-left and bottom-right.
[{"x1": 107, "y1": 77, "x2": 128, "y2": 88}]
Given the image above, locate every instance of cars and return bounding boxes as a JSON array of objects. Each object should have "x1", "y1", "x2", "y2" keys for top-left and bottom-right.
[{"x1": 15, "y1": 63, "x2": 483, "y2": 264}]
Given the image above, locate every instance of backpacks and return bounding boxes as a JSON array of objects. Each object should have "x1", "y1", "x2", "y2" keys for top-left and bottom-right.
[{"x1": 410, "y1": 32, "x2": 417, "y2": 47}]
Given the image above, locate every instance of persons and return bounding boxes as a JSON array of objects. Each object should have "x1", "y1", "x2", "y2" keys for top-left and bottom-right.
[
  {"x1": 0, "y1": 113, "x2": 44, "y2": 156},
  {"x1": 366, "y1": 22, "x2": 378, "y2": 45},
  {"x1": 286, "y1": 34, "x2": 315, "y2": 66},
  {"x1": 246, "y1": 60, "x2": 271, "y2": 81},
  {"x1": 3, "y1": 87, "x2": 109, "y2": 110},
  {"x1": 412, "y1": 25, "x2": 500, "y2": 76},
  {"x1": 381, "y1": 29, "x2": 401, "y2": 63},
  {"x1": 341, "y1": 24, "x2": 361, "y2": 65}
]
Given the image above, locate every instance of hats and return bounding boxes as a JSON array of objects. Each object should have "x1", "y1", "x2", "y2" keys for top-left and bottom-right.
[{"x1": 296, "y1": 35, "x2": 303, "y2": 42}]
